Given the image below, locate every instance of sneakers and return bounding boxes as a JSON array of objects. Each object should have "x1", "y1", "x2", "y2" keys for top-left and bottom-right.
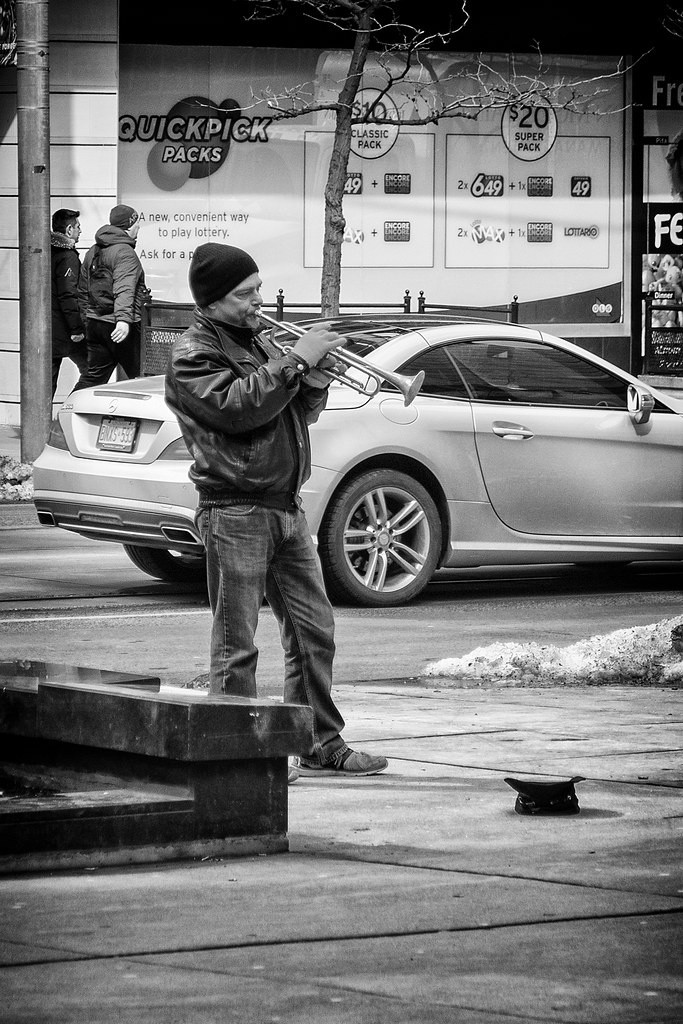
[{"x1": 289, "y1": 748, "x2": 388, "y2": 778}]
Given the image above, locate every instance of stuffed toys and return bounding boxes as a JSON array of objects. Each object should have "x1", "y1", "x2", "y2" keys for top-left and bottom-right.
[{"x1": 641, "y1": 254, "x2": 683, "y2": 328}]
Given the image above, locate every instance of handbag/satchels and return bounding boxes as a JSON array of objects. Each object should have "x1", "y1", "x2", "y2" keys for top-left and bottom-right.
[{"x1": 88, "y1": 268, "x2": 115, "y2": 315}]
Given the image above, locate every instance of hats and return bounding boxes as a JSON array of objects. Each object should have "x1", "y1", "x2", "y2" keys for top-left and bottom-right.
[
  {"x1": 109, "y1": 205, "x2": 138, "y2": 229},
  {"x1": 503, "y1": 775, "x2": 586, "y2": 816},
  {"x1": 188, "y1": 242, "x2": 257, "y2": 310}
]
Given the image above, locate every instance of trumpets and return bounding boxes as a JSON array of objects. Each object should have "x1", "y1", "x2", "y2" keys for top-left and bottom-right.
[{"x1": 252, "y1": 307, "x2": 426, "y2": 408}]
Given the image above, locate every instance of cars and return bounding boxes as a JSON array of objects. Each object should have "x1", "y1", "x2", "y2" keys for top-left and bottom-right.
[{"x1": 33, "y1": 311, "x2": 683, "y2": 609}]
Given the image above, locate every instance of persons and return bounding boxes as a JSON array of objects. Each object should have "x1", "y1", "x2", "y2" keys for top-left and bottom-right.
[
  {"x1": 49, "y1": 203, "x2": 154, "y2": 406},
  {"x1": 164, "y1": 240, "x2": 388, "y2": 783}
]
